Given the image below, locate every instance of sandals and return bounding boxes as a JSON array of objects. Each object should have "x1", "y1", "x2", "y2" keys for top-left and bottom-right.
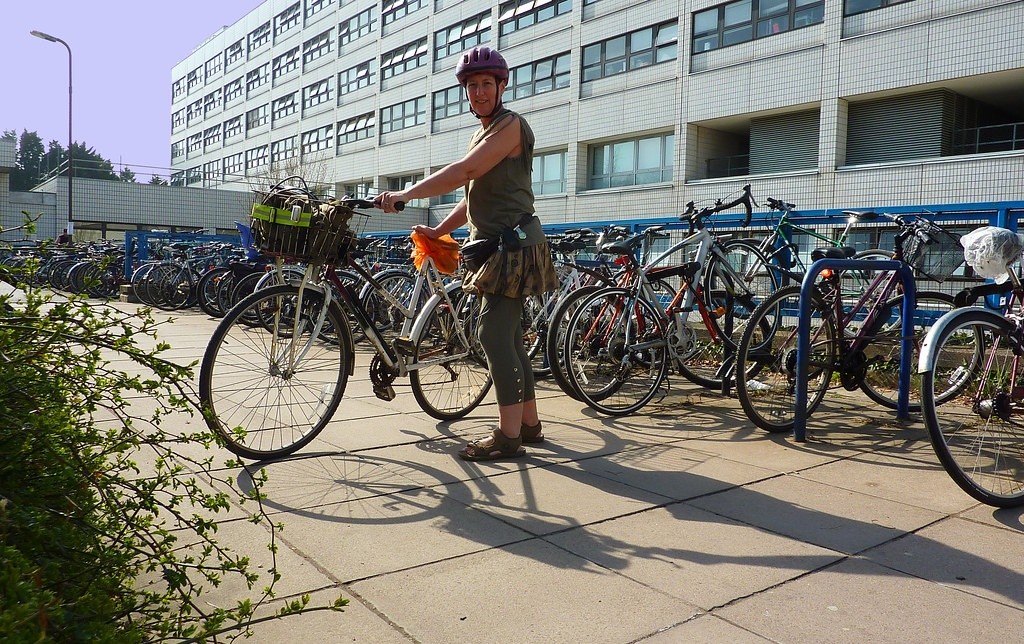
[{"x1": 457, "y1": 420, "x2": 544, "y2": 461}]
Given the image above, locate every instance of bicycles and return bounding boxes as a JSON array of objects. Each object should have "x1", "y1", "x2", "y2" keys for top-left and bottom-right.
[{"x1": 0, "y1": 176, "x2": 1024, "y2": 510}]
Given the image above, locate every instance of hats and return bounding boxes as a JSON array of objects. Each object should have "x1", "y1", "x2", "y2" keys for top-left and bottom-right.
[{"x1": 455, "y1": 46, "x2": 510, "y2": 87}]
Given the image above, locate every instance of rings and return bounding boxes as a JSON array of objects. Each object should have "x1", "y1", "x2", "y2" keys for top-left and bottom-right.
[{"x1": 384, "y1": 203, "x2": 388, "y2": 206}]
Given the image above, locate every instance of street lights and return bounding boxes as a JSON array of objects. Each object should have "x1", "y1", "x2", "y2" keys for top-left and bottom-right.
[{"x1": 29, "y1": 29, "x2": 73, "y2": 243}]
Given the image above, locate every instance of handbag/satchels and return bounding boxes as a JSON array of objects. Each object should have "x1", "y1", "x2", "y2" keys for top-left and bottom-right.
[{"x1": 459, "y1": 213, "x2": 534, "y2": 274}]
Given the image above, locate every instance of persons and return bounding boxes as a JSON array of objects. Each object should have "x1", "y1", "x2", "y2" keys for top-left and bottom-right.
[{"x1": 374, "y1": 47, "x2": 560, "y2": 462}]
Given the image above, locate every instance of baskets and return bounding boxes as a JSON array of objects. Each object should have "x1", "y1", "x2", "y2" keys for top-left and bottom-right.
[
  {"x1": 251, "y1": 189, "x2": 370, "y2": 271},
  {"x1": 906, "y1": 214, "x2": 964, "y2": 283}
]
